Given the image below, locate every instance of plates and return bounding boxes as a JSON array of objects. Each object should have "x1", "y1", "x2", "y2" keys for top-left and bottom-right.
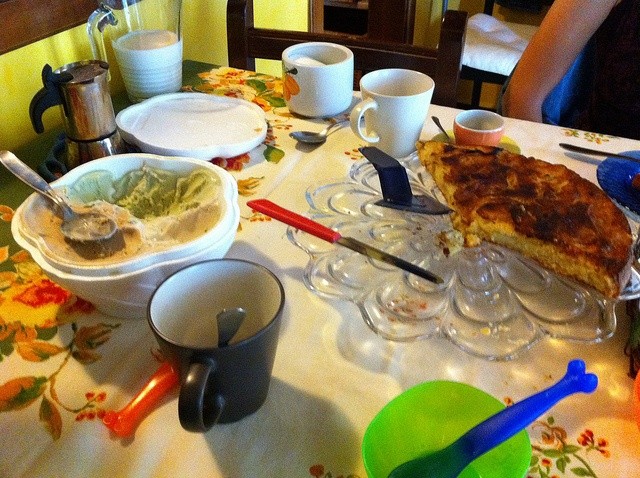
[
  {"x1": 116, "y1": 92, "x2": 268, "y2": 160},
  {"x1": 596, "y1": 149, "x2": 640, "y2": 216},
  {"x1": 287, "y1": 152, "x2": 640, "y2": 359}
]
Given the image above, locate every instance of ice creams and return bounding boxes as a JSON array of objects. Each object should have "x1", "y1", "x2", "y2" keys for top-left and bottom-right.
[{"x1": 18, "y1": 162, "x2": 223, "y2": 265}]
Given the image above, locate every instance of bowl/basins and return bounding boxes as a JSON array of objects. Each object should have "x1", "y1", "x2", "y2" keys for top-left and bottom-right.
[{"x1": 11, "y1": 153, "x2": 240, "y2": 317}]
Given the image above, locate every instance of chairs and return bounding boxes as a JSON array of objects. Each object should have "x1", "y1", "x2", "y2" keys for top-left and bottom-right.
[{"x1": 227, "y1": 0, "x2": 469, "y2": 106}]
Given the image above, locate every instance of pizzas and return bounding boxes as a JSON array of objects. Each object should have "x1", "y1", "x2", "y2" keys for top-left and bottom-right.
[{"x1": 416, "y1": 141, "x2": 633, "y2": 300}]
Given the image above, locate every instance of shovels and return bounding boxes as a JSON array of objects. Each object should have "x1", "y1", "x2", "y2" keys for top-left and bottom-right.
[{"x1": 356, "y1": 145, "x2": 457, "y2": 219}]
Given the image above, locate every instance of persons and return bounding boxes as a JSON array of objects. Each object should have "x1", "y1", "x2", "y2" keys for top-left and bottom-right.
[{"x1": 500, "y1": 0, "x2": 639, "y2": 142}]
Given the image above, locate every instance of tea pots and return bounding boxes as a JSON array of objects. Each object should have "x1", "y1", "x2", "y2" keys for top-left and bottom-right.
[
  {"x1": 84, "y1": 1, "x2": 185, "y2": 96},
  {"x1": 27, "y1": 59, "x2": 128, "y2": 169}
]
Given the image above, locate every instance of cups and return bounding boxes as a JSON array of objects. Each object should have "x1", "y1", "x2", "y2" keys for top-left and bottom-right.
[
  {"x1": 146, "y1": 259, "x2": 286, "y2": 432},
  {"x1": 280, "y1": 43, "x2": 353, "y2": 116},
  {"x1": 362, "y1": 380, "x2": 533, "y2": 474},
  {"x1": 453, "y1": 109, "x2": 506, "y2": 147},
  {"x1": 349, "y1": 69, "x2": 435, "y2": 157}
]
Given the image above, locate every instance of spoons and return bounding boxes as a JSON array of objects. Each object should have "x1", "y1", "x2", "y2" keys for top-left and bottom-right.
[
  {"x1": 1, "y1": 150, "x2": 119, "y2": 242},
  {"x1": 287, "y1": 119, "x2": 355, "y2": 143}
]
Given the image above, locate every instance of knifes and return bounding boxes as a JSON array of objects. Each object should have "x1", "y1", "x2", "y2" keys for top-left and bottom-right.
[{"x1": 246, "y1": 198, "x2": 445, "y2": 287}]
